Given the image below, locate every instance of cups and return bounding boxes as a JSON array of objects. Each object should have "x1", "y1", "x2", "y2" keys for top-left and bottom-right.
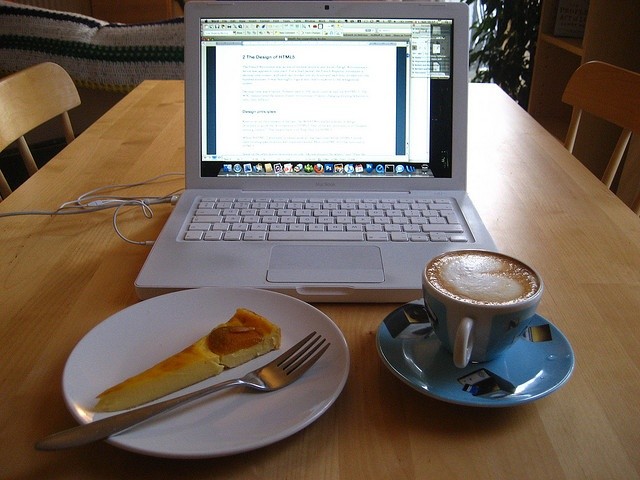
[{"x1": 422, "y1": 249, "x2": 543, "y2": 369}]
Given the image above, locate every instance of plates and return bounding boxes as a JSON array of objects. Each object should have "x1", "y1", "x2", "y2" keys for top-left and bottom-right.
[
  {"x1": 376, "y1": 297, "x2": 575, "y2": 408},
  {"x1": 61, "y1": 286, "x2": 350, "y2": 459}
]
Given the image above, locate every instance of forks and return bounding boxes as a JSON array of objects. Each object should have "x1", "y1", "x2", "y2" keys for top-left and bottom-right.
[{"x1": 35, "y1": 330, "x2": 331, "y2": 451}]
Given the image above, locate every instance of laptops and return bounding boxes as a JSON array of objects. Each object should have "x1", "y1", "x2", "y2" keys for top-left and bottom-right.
[{"x1": 131, "y1": 0, "x2": 499, "y2": 305}]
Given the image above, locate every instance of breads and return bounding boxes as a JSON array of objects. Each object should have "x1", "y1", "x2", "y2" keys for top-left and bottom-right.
[{"x1": 94, "y1": 308, "x2": 282, "y2": 412}]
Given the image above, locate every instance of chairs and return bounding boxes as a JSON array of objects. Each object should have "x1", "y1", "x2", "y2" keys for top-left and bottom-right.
[
  {"x1": 558, "y1": 58, "x2": 639, "y2": 190},
  {"x1": 0, "y1": 60, "x2": 82, "y2": 197}
]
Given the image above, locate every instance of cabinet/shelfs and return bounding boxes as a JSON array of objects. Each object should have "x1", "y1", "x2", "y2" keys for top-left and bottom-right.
[{"x1": 525, "y1": 0, "x2": 640, "y2": 211}]
